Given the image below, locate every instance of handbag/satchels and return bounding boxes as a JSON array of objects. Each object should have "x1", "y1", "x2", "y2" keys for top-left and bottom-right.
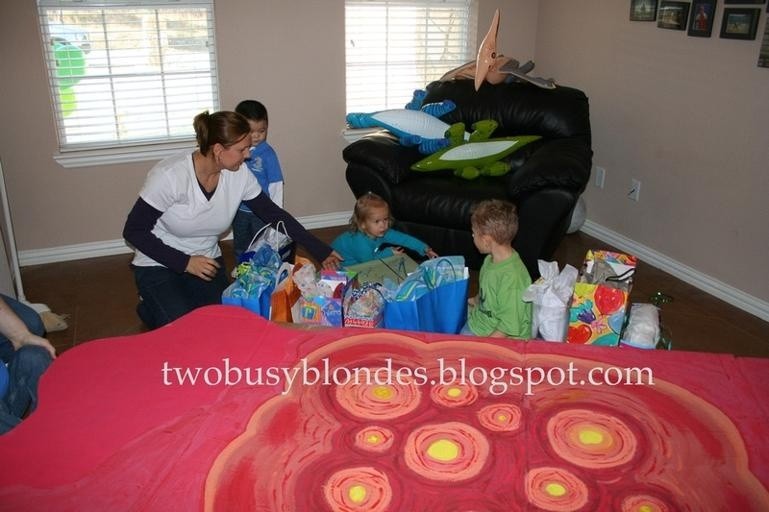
[
  {"x1": 299, "y1": 268, "x2": 358, "y2": 326},
  {"x1": 220, "y1": 220, "x2": 299, "y2": 321},
  {"x1": 561, "y1": 248, "x2": 637, "y2": 347},
  {"x1": 619, "y1": 301, "x2": 662, "y2": 350},
  {"x1": 343, "y1": 282, "x2": 390, "y2": 327},
  {"x1": 381, "y1": 252, "x2": 472, "y2": 333},
  {"x1": 270, "y1": 262, "x2": 304, "y2": 323}
]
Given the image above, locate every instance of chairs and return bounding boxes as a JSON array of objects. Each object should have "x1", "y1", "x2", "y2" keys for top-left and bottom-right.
[{"x1": 335, "y1": 69, "x2": 592, "y2": 268}]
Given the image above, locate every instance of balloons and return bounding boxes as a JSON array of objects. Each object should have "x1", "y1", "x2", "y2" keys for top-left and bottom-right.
[{"x1": 50, "y1": 36, "x2": 86, "y2": 118}]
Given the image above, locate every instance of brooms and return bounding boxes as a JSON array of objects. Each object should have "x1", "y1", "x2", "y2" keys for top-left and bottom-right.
[{"x1": 0, "y1": 151, "x2": 70, "y2": 332}]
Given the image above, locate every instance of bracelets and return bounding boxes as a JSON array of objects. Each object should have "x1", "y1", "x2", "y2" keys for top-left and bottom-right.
[{"x1": 425, "y1": 245, "x2": 433, "y2": 255}]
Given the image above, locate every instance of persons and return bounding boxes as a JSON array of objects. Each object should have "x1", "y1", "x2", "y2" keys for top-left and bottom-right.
[
  {"x1": 122, "y1": 112, "x2": 344, "y2": 331},
  {"x1": 456, "y1": 200, "x2": 534, "y2": 339},
  {"x1": 228, "y1": 99, "x2": 286, "y2": 280},
  {"x1": 330, "y1": 191, "x2": 438, "y2": 270},
  {"x1": 694, "y1": 6, "x2": 708, "y2": 31},
  {"x1": 0, "y1": 294, "x2": 57, "y2": 436},
  {"x1": 347, "y1": 89, "x2": 472, "y2": 155}
]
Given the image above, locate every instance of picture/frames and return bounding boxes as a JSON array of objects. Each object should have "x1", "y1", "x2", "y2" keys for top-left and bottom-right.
[{"x1": 627, "y1": 0, "x2": 769, "y2": 44}]
